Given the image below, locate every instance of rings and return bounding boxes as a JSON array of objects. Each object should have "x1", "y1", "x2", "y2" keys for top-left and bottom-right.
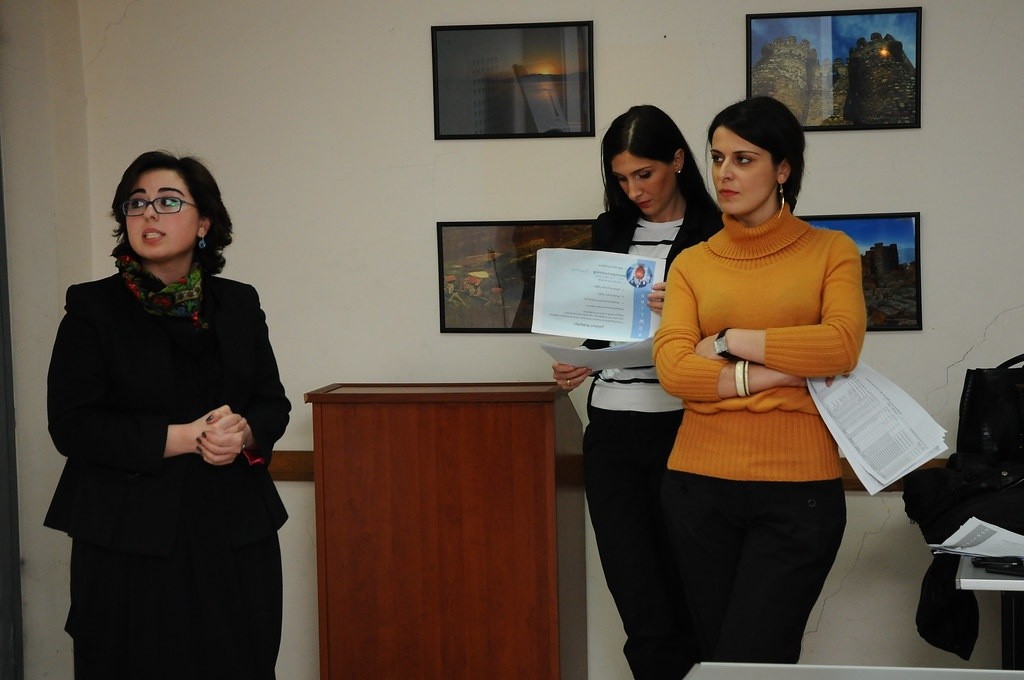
[{"x1": 567, "y1": 380, "x2": 570, "y2": 385}]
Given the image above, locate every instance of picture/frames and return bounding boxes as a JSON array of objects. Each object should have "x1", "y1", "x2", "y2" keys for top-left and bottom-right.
[
  {"x1": 437, "y1": 219, "x2": 595, "y2": 335},
  {"x1": 794, "y1": 211, "x2": 923, "y2": 332},
  {"x1": 745, "y1": 6, "x2": 923, "y2": 133},
  {"x1": 430, "y1": 20, "x2": 596, "y2": 139}
]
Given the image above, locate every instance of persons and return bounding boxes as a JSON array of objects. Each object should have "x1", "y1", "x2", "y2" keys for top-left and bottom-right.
[
  {"x1": 550, "y1": 105, "x2": 725, "y2": 680},
  {"x1": 653, "y1": 99, "x2": 867, "y2": 663},
  {"x1": 44, "y1": 151, "x2": 292, "y2": 680}
]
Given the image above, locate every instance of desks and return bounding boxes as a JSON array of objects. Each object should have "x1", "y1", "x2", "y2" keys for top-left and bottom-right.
[{"x1": 303, "y1": 382, "x2": 588, "y2": 680}]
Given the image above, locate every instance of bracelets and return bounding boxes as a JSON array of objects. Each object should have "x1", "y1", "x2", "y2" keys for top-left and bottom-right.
[
  {"x1": 736, "y1": 361, "x2": 750, "y2": 397},
  {"x1": 242, "y1": 441, "x2": 247, "y2": 449}
]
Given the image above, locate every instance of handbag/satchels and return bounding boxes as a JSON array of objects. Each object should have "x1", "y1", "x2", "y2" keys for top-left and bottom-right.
[{"x1": 957, "y1": 353, "x2": 1024, "y2": 474}]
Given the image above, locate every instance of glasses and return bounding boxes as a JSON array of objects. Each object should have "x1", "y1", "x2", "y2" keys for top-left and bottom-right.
[{"x1": 123, "y1": 196, "x2": 199, "y2": 217}]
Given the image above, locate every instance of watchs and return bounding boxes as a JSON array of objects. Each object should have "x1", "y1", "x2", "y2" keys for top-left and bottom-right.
[{"x1": 714, "y1": 328, "x2": 742, "y2": 360}]
